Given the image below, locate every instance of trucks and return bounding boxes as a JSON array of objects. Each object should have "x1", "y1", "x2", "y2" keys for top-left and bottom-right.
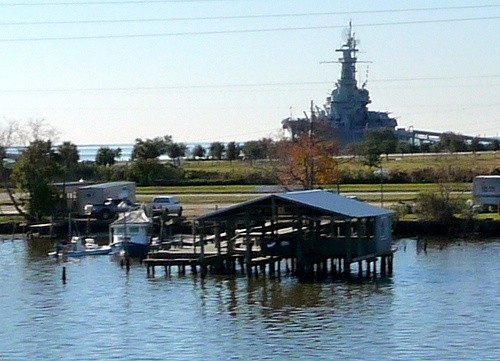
[{"x1": 77, "y1": 182, "x2": 136, "y2": 217}]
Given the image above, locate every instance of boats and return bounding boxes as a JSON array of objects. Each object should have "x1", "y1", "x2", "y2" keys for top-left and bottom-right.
[
  {"x1": 280, "y1": 16, "x2": 415, "y2": 145},
  {"x1": 108, "y1": 204, "x2": 153, "y2": 256},
  {"x1": 46, "y1": 235, "x2": 170, "y2": 257}
]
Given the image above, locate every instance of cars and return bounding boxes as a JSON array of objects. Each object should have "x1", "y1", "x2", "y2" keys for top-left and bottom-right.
[
  {"x1": 90, "y1": 199, "x2": 147, "y2": 221},
  {"x1": 150, "y1": 196, "x2": 183, "y2": 217}
]
